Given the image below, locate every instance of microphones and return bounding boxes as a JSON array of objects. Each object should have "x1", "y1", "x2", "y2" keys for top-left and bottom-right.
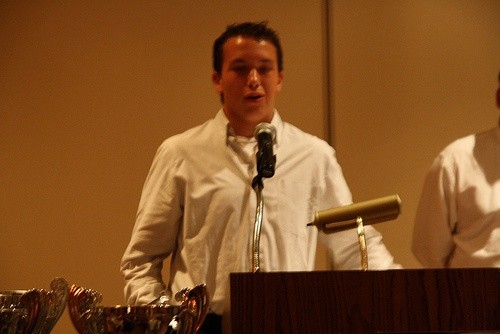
[
  {"x1": 307, "y1": 193, "x2": 403, "y2": 235},
  {"x1": 252, "y1": 121, "x2": 277, "y2": 178}
]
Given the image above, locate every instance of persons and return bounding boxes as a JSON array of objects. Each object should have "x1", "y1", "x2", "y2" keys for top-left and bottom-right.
[
  {"x1": 411, "y1": 70, "x2": 500, "y2": 268},
  {"x1": 120, "y1": 22, "x2": 404, "y2": 334}
]
